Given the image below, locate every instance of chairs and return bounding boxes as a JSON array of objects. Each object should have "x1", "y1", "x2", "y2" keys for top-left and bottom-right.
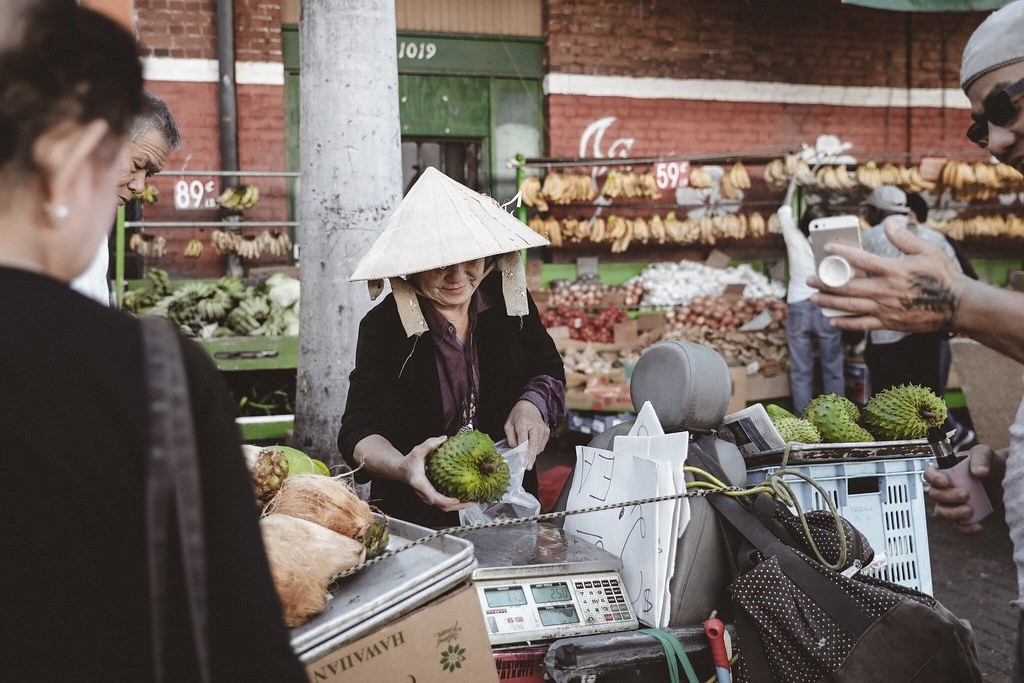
[{"x1": 548, "y1": 338, "x2": 746, "y2": 628}]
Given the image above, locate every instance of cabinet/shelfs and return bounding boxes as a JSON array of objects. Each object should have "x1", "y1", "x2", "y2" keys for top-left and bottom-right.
[{"x1": 111, "y1": 278, "x2": 299, "y2": 441}]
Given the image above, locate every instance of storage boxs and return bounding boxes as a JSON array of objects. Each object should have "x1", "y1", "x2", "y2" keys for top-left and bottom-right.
[
  {"x1": 300, "y1": 575, "x2": 501, "y2": 683},
  {"x1": 538, "y1": 286, "x2": 792, "y2": 415},
  {"x1": 745, "y1": 439, "x2": 937, "y2": 600}
]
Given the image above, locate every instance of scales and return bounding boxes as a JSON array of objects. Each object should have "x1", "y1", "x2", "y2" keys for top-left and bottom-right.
[{"x1": 430, "y1": 522, "x2": 640, "y2": 647}]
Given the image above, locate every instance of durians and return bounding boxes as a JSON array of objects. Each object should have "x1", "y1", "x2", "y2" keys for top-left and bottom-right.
[
  {"x1": 428, "y1": 430, "x2": 511, "y2": 501},
  {"x1": 767, "y1": 385, "x2": 948, "y2": 444}
]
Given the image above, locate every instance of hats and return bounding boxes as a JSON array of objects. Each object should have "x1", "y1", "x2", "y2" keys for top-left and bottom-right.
[
  {"x1": 348, "y1": 165, "x2": 552, "y2": 338},
  {"x1": 861, "y1": 185, "x2": 912, "y2": 214},
  {"x1": 959, "y1": 0, "x2": 1024, "y2": 93}
]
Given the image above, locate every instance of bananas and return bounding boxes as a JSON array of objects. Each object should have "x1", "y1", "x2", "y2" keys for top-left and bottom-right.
[
  {"x1": 124, "y1": 183, "x2": 292, "y2": 337},
  {"x1": 522, "y1": 158, "x2": 1024, "y2": 253}
]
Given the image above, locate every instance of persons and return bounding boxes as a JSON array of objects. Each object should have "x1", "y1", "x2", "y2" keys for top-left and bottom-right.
[
  {"x1": 904, "y1": 193, "x2": 979, "y2": 394},
  {"x1": 101, "y1": 92, "x2": 188, "y2": 209},
  {"x1": 776, "y1": 170, "x2": 845, "y2": 418},
  {"x1": 860, "y1": 186, "x2": 964, "y2": 401},
  {"x1": 805, "y1": 1, "x2": 1023, "y2": 683},
  {"x1": 338, "y1": 166, "x2": 568, "y2": 527},
  {"x1": 0, "y1": 0, "x2": 314, "y2": 683}
]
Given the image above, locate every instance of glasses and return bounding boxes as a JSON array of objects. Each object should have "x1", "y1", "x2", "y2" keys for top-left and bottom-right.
[{"x1": 966, "y1": 78, "x2": 1024, "y2": 149}]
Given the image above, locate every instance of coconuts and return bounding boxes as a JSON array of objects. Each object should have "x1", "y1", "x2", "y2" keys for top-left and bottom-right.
[
  {"x1": 269, "y1": 475, "x2": 389, "y2": 556},
  {"x1": 261, "y1": 515, "x2": 366, "y2": 583}
]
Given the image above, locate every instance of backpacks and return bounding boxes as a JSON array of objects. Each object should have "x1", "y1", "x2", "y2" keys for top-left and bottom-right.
[{"x1": 681, "y1": 440, "x2": 990, "y2": 683}]
